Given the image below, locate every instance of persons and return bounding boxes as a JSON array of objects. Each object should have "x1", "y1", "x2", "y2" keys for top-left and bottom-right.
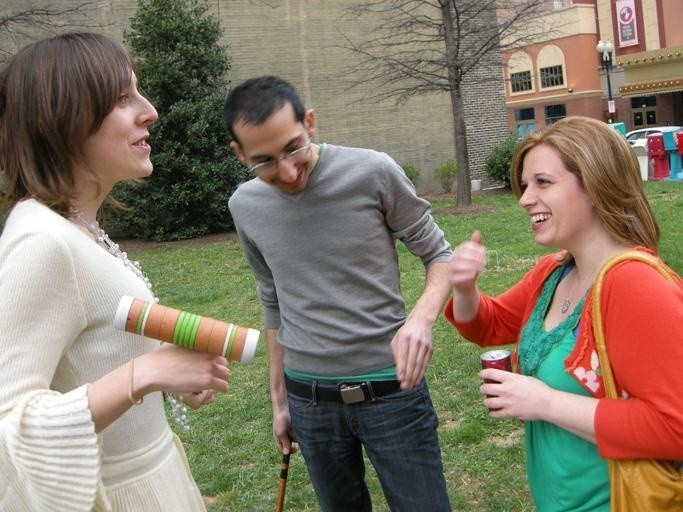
[
  {"x1": 0, "y1": 30, "x2": 233, "y2": 512},
  {"x1": 441, "y1": 118, "x2": 683, "y2": 512},
  {"x1": 223, "y1": 75, "x2": 454, "y2": 512}
]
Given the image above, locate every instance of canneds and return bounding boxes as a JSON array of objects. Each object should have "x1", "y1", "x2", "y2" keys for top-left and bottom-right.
[{"x1": 481, "y1": 350, "x2": 511, "y2": 413}]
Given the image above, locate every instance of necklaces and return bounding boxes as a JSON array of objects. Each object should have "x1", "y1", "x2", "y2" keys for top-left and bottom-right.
[
  {"x1": 73, "y1": 208, "x2": 189, "y2": 432},
  {"x1": 560, "y1": 274, "x2": 584, "y2": 313}
]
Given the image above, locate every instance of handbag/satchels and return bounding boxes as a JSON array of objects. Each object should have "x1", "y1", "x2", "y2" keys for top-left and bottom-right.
[{"x1": 597, "y1": 451, "x2": 683, "y2": 512}]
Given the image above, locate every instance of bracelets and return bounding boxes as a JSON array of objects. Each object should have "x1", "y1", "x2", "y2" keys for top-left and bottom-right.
[{"x1": 128, "y1": 358, "x2": 144, "y2": 407}]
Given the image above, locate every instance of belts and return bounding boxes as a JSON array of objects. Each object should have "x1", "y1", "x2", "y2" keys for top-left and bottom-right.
[{"x1": 285, "y1": 376, "x2": 398, "y2": 405}]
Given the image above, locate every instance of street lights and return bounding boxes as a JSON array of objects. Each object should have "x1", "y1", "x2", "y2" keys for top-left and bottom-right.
[{"x1": 596, "y1": 38, "x2": 615, "y2": 124}]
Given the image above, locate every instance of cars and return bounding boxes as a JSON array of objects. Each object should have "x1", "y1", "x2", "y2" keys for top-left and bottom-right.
[{"x1": 624, "y1": 125, "x2": 680, "y2": 144}]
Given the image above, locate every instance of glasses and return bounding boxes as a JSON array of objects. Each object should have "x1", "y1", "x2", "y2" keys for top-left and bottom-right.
[{"x1": 245, "y1": 137, "x2": 313, "y2": 177}]
